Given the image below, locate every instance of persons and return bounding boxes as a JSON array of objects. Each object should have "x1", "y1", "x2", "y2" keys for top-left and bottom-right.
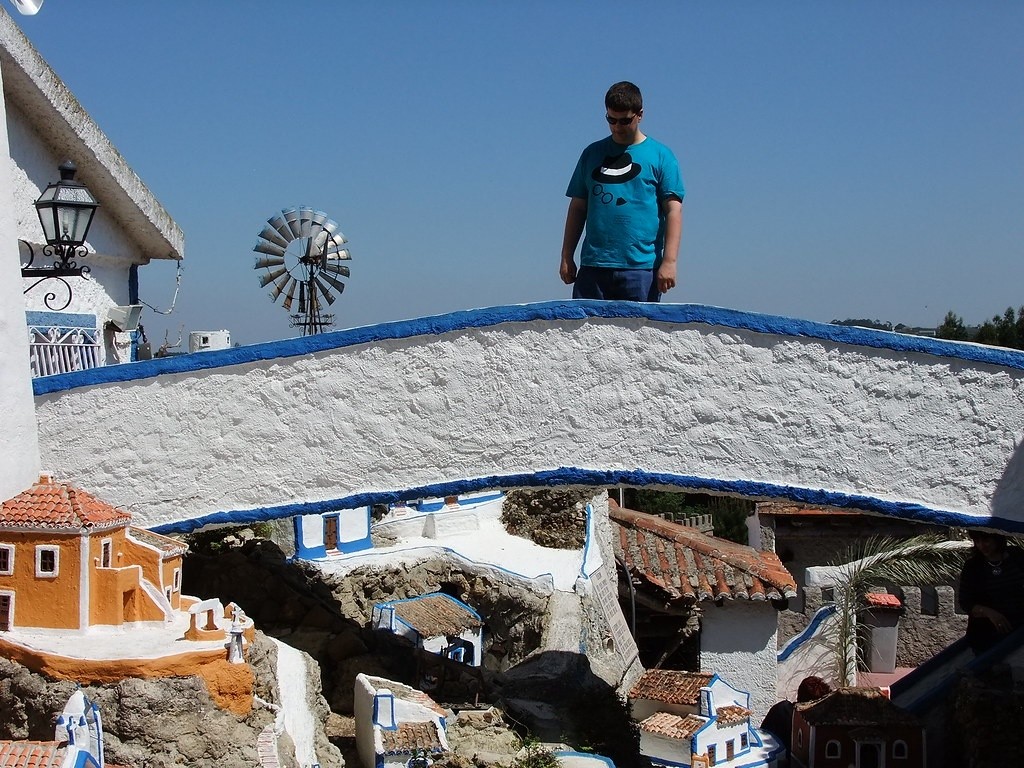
[
  {"x1": 559, "y1": 81, "x2": 686, "y2": 304},
  {"x1": 957, "y1": 527, "x2": 1024, "y2": 638}
]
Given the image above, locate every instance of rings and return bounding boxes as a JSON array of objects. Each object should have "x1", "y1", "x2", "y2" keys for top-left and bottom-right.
[{"x1": 998, "y1": 623, "x2": 1002, "y2": 626}]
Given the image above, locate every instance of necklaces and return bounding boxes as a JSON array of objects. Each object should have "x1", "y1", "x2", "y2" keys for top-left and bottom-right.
[{"x1": 984, "y1": 548, "x2": 1005, "y2": 576}]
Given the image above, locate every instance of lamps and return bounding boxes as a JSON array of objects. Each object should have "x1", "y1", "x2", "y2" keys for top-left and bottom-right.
[
  {"x1": 20, "y1": 159, "x2": 102, "y2": 311},
  {"x1": 106, "y1": 303, "x2": 143, "y2": 332}
]
